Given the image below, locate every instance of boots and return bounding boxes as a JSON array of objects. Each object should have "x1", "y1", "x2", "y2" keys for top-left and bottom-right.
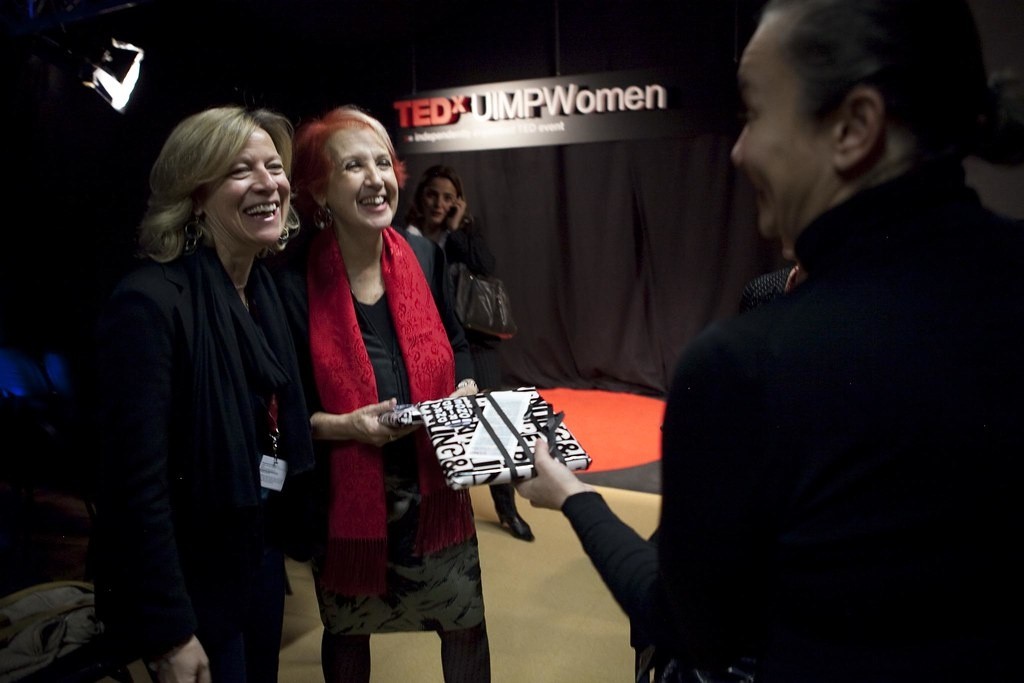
[{"x1": 488, "y1": 484, "x2": 535, "y2": 542}]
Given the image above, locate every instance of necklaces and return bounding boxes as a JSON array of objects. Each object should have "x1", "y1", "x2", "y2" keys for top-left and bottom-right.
[{"x1": 246, "y1": 300, "x2": 248, "y2": 307}]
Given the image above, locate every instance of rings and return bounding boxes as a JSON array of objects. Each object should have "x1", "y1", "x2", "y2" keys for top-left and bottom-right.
[{"x1": 389, "y1": 434, "x2": 393, "y2": 442}]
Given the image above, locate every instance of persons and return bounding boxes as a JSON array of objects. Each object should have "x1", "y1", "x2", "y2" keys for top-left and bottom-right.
[
  {"x1": 287, "y1": 103, "x2": 493, "y2": 683},
  {"x1": 96, "y1": 103, "x2": 308, "y2": 683},
  {"x1": 403, "y1": 163, "x2": 536, "y2": 544},
  {"x1": 515, "y1": 0, "x2": 1024, "y2": 682}
]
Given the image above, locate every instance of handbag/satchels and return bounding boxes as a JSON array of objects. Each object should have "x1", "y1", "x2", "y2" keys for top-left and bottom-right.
[{"x1": 448, "y1": 256, "x2": 518, "y2": 341}]
